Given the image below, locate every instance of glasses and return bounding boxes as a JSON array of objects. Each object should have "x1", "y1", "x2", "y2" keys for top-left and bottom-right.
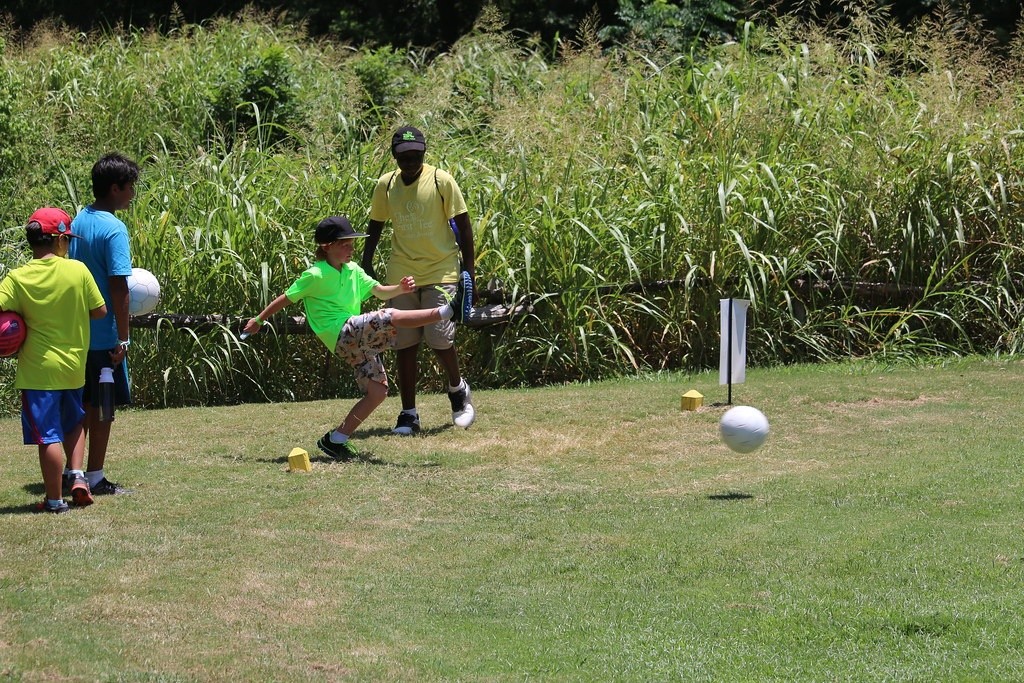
[{"x1": 52, "y1": 233, "x2": 72, "y2": 245}]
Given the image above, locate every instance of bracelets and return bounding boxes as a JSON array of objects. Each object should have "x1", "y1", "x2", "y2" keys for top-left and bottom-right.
[
  {"x1": 255, "y1": 315, "x2": 267, "y2": 327},
  {"x1": 116, "y1": 338, "x2": 130, "y2": 349}
]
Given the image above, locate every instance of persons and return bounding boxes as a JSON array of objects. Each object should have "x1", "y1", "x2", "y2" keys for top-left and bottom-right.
[
  {"x1": 360, "y1": 126, "x2": 476, "y2": 436},
  {"x1": 0, "y1": 207, "x2": 108, "y2": 513},
  {"x1": 243, "y1": 217, "x2": 473, "y2": 462},
  {"x1": 60, "y1": 151, "x2": 140, "y2": 494}
]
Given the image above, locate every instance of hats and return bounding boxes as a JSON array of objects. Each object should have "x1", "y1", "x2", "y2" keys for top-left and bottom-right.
[
  {"x1": 315, "y1": 217, "x2": 370, "y2": 244},
  {"x1": 26, "y1": 208, "x2": 84, "y2": 240},
  {"x1": 392, "y1": 127, "x2": 426, "y2": 153}
]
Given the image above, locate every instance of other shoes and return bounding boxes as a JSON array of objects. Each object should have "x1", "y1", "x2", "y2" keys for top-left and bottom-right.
[
  {"x1": 92, "y1": 477, "x2": 125, "y2": 495},
  {"x1": 62, "y1": 472, "x2": 85, "y2": 485}
]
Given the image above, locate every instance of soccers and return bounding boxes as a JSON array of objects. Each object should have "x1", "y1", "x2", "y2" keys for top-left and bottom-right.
[{"x1": 720, "y1": 406, "x2": 771, "y2": 455}]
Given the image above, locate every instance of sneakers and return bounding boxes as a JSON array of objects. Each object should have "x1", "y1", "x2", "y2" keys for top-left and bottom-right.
[
  {"x1": 392, "y1": 411, "x2": 419, "y2": 434},
  {"x1": 448, "y1": 380, "x2": 476, "y2": 428},
  {"x1": 450, "y1": 271, "x2": 472, "y2": 324},
  {"x1": 69, "y1": 472, "x2": 94, "y2": 505},
  {"x1": 37, "y1": 498, "x2": 69, "y2": 512},
  {"x1": 317, "y1": 429, "x2": 358, "y2": 462}
]
